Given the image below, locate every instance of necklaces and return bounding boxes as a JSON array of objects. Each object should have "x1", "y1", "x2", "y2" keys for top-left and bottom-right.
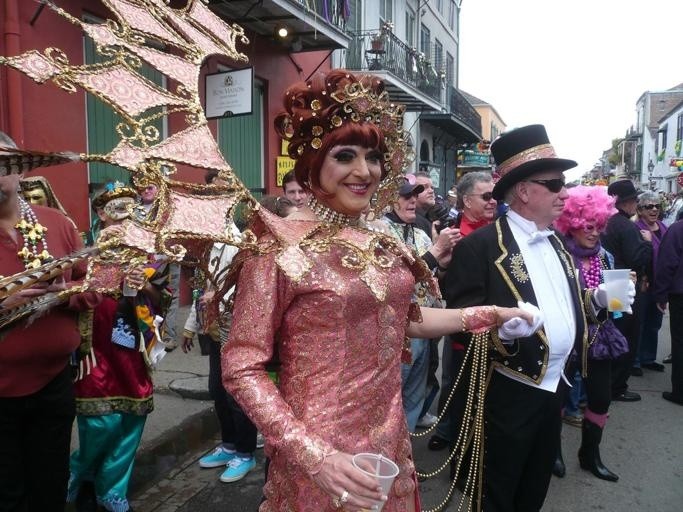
[{"x1": 306, "y1": 193, "x2": 360, "y2": 225}]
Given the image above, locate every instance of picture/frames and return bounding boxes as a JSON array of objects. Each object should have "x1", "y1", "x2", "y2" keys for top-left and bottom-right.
[{"x1": 204, "y1": 65, "x2": 254, "y2": 120}]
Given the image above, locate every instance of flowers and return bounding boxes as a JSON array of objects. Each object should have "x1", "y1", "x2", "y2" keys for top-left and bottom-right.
[{"x1": 373, "y1": 30, "x2": 390, "y2": 43}]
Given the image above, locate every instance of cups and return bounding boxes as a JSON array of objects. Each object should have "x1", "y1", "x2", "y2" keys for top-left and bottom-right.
[
  {"x1": 122, "y1": 277, "x2": 138, "y2": 297},
  {"x1": 353, "y1": 453, "x2": 399, "y2": 511},
  {"x1": 602, "y1": 269, "x2": 633, "y2": 311}
]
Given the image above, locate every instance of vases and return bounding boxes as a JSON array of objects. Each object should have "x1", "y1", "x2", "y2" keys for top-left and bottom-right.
[{"x1": 372, "y1": 40, "x2": 384, "y2": 50}]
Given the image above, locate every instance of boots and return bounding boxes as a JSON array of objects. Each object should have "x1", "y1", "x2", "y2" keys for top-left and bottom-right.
[
  {"x1": 578, "y1": 418, "x2": 618, "y2": 481},
  {"x1": 552, "y1": 439, "x2": 565, "y2": 477}
]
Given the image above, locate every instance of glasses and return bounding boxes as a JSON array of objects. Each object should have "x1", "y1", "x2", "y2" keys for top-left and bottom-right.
[
  {"x1": 469, "y1": 192, "x2": 493, "y2": 201},
  {"x1": 520, "y1": 177, "x2": 565, "y2": 192},
  {"x1": 637, "y1": 204, "x2": 660, "y2": 209},
  {"x1": 578, "y1": 224, "x2": 607, "y2": 233}
]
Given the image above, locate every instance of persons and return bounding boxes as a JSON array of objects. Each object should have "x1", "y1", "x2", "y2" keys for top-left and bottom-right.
[
  {"x1": 1, "y1": 131, "x2": 308, "y2": 512},
  {"x1": 221, "y1": 70, "x2": 534, "y2": 512},
  {"x1": 441, "y1": 124, "x2": 636, "y2": 512},
  {"x1": 376, "y1": 171, "x2": 497, "y2": 452},
  {"x1": 553, "y1": 179, "x2": 683, "y2": 482}
]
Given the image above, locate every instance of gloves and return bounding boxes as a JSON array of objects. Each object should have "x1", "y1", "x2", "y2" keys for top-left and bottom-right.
[
  {"x1": 594, "y1": 279, "x2": 636, "y2": 314},
  {"x1": 497, "y1": 301, "x2": 545, "y2": 340}
]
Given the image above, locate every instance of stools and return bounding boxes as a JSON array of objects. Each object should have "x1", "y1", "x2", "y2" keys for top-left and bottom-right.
[{"x1": 368, "y1": 50, "x2": 386, "y2": 70}]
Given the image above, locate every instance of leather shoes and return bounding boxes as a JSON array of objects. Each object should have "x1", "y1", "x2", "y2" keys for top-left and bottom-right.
[
  {"x1": 562, "y1": 355, "x2": 683, "y2": 428},
  {"x1": 429, "y1": 435, "x2": 449, "y2": 450}
]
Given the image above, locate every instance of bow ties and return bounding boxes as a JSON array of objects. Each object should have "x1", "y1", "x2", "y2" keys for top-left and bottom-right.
[{"x1": 507, "y1": 210, "x2": 554, "y2": 245}]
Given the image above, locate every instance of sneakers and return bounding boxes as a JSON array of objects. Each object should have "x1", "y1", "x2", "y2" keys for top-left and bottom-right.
[
  {"x1": 220, "y1": 456, "x2": 256, "y2": 483},
  {"x1": 416, "y1": 413, "x2": 438, "y2": 428},
  {"x1": 199, "y1": 447, "x2": 237, "y2": 468}
]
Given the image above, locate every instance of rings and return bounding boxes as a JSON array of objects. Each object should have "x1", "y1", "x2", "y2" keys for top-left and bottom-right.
[{"x1": 341, "y1": 491, "x2": 348, "y2": 502}]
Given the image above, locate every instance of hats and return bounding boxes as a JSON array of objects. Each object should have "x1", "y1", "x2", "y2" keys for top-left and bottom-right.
[
  {"x1": 491, "y1": 124, "x2": 577, "y2": 200},
  {"x1": 608, "y1": 180, "x2": 642, "y2": 202},
  {"x1": 388, "y1": 178, "x2": 424, "y2": 196}
]
